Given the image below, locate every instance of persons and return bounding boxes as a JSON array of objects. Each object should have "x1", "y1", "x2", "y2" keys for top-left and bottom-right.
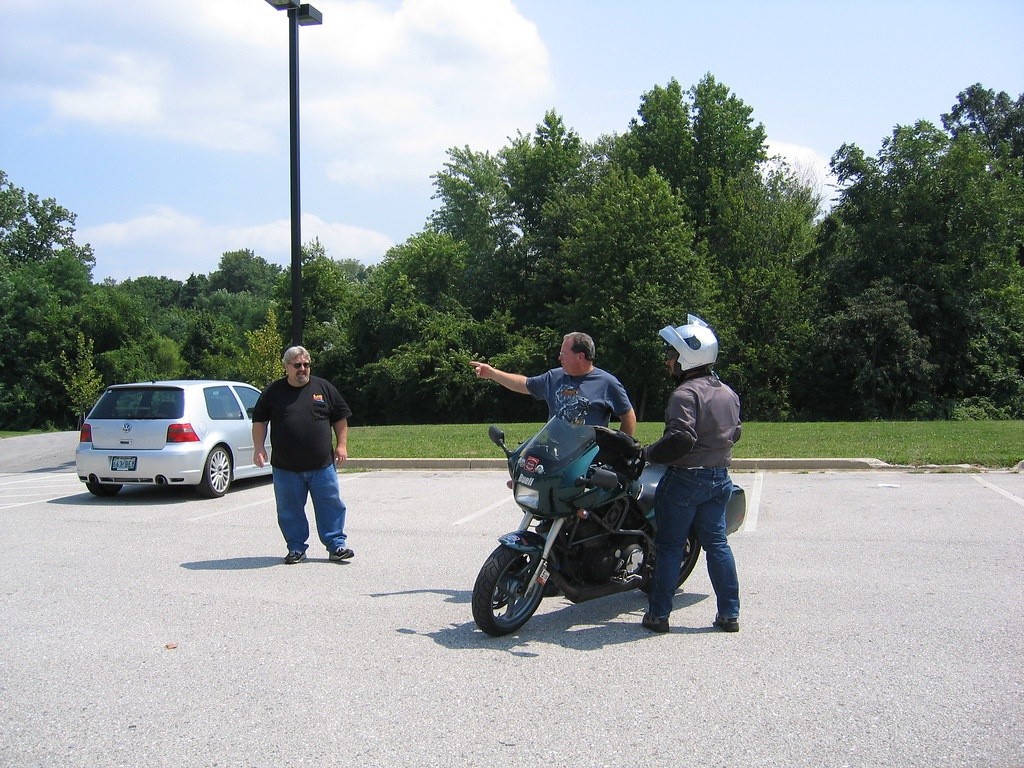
[
  {"x1": 251, "y1": 345, "x2": 354, "y2": 564},
  {"x1": 469, "y1": 313, "x2": 740, "y2": 633}
]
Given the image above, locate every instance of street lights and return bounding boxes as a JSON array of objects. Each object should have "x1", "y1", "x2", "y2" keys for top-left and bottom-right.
[{"x1": 263, "y1": 0, "x2": 324, "y2": 348}]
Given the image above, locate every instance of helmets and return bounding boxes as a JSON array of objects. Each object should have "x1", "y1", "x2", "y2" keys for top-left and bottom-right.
[{"x1": 658, "y1": 312, "x2": 719, "y2": 371}]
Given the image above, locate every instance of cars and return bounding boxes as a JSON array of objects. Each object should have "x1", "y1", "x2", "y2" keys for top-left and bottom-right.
[{"x1": 74, "y1": 378, "x2": 273, "y2": 500}]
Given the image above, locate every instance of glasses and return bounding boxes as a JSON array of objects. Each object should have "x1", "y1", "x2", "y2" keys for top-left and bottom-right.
[
  {"x1": 663, "y1": 346, "x2": 677, "y2": 360},
  {"x1": 288, "y1": 363, "x2": 312, "y2": 369}
]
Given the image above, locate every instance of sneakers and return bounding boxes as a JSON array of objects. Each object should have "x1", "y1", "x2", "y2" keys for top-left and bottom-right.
[
  {"x1": 329, "y1": 547, "x2": 355, "y2": 561},
  {"x1": 284, "y1": 550, "x2": 307, "y2": 564}
]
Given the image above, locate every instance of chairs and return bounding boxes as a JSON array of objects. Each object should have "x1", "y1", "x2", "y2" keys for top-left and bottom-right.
[{"x1": 157, "y1": 401, "x2": 178, "y2": 418}]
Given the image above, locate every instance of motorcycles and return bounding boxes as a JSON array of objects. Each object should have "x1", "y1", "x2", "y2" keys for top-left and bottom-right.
[{"x1": 471, "y1": 414, "x2": 748, "y2": 639}]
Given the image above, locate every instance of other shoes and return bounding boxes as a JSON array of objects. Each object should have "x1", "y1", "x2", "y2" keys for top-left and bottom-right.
[
  {"x1": 642, "y1": 612, "x2": 670, "y2": 632},
  {"x1": 715, "y1": 613, "x2": 739, "y2": 632}
]
